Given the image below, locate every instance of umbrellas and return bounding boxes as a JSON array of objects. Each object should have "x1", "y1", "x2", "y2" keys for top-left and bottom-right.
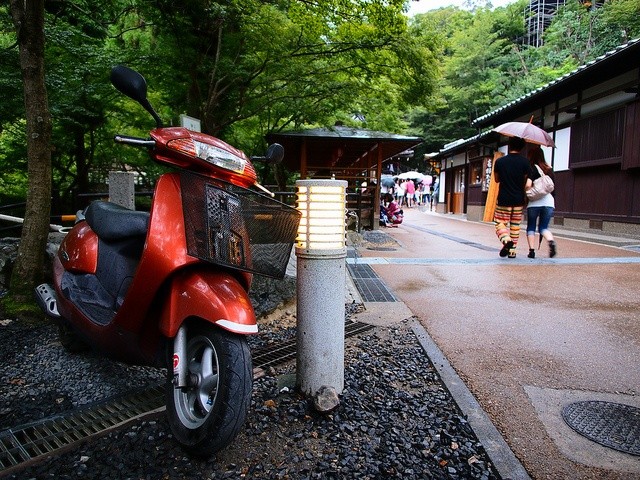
[
  {"x1": 380, "y1": 177, "x2": 395, "y2": 187},
  {"x1": 394, "y1": 170, "x2": 426, "y2": 179},
  {"x1": 421, "y1": 175, "x2": 432, "y2": 185},
  {"x1": 490, "y1": 115, "x2": 556, "y2": 147}
]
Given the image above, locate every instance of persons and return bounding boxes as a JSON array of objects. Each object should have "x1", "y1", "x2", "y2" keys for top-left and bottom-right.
[
  {"x1": 415, "y1": 183, "x2": 421, "y2": 202},
  {"x1": 387, "y1": 186, "x2": 393, "y2": 195},
  {"x1": 393, "y1": 177, "x2": 404, "y2": 202},
  {"x1": 419, "y1": 183, "x2": 425, "y2": 192},
  {"x1": 524, "y1": 146, "x2": 558, "y2": 258},
  {"x1": 382, "y1": 193, "x2": 405, "y2": 228},
  {"x1": 405, "y1": 178, "x2": 415, "y2": 208},
  {"x1": 395, "y1": 179, "x2": 407, "y2": 207},
  {"x1": 423, "y1": 184, "x2": 431, "y2": 208},
  {"x1": 493, "y1": 136, "x2": 531, "y2": 259}
]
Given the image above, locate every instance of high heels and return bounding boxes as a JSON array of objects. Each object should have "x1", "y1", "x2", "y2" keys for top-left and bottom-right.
[
  {"x1": 528, "y1": 249, "x2": 535, "y2": 258},
  {"x1": 548, "y1": 240, "x2": 557, "y2": 258}
]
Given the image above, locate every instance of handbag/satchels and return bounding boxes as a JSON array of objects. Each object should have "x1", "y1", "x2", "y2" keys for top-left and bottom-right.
[{"x1": 527, "y1": 176, "x2": 554, "y2": 202}]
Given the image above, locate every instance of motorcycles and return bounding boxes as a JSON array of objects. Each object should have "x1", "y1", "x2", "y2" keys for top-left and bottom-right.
[{"x1": 34, "y1": 65, "x2": 303, "y2": 460}]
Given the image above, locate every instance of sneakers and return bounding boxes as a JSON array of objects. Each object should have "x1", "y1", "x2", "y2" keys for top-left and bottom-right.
[
  {"x1": 509, "y1": 253, "x2": 516, "y2": 258},
  {"x1": 499, "y1": 241, "x2": 513, "y2": 257}
]
